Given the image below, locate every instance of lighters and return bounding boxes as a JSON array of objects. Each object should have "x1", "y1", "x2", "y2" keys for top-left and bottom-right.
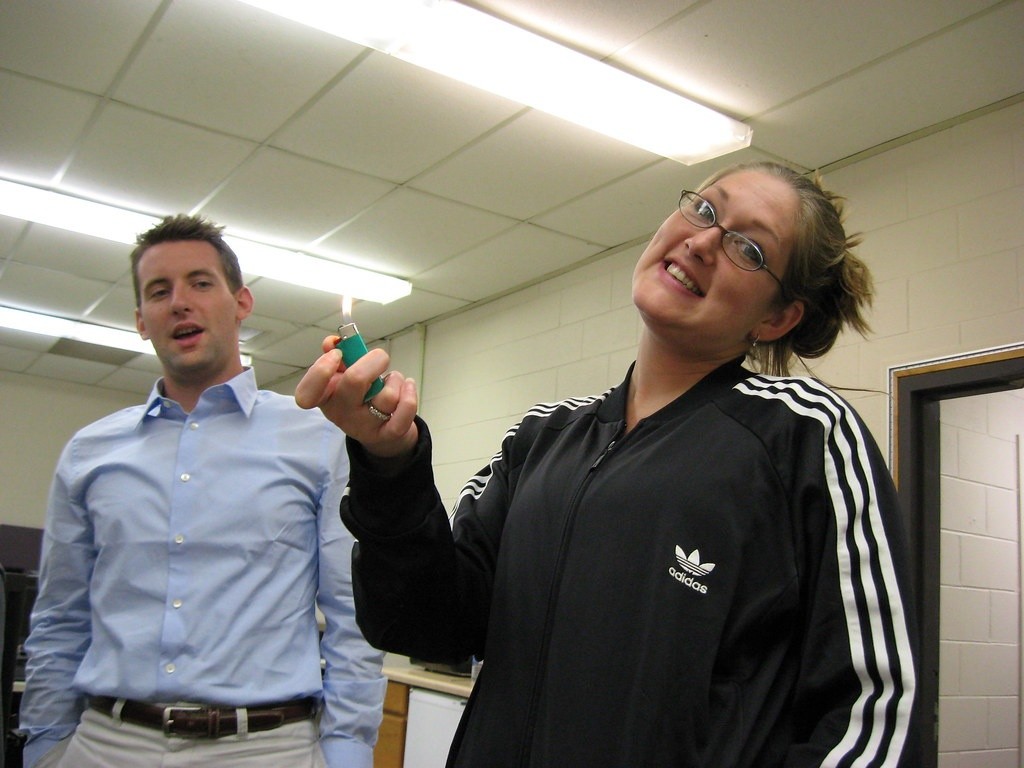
[{"x1": 336, "y1": 320, "x2": 385, "y2": 405}]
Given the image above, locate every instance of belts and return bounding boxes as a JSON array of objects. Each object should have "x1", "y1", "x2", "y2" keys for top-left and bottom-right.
[{"x1": 88, "y1": 694, "x2": 315, "y2": 739}]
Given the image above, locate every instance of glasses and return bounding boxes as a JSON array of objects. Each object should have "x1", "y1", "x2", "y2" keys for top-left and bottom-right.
[{"x1": 679, "y1": 190, "x2": 784, "y2": 291}]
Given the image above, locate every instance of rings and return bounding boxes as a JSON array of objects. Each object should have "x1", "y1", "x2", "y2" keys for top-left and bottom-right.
[{"x1": 368, "y1": 402, "x2": 391, "y2": 421}]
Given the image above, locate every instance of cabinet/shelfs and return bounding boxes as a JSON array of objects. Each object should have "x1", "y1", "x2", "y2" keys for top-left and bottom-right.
[{"x1": 321, "y1": 659, "x2": 478, "y2": 768}]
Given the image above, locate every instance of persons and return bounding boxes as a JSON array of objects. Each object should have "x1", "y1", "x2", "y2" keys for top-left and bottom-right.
[
  {"x1": 0, "y1": 215, "x2": 389, "y2": 768},
  {"x1": 295, "y1": 162, "x2": 919, "y2": 768}
]
